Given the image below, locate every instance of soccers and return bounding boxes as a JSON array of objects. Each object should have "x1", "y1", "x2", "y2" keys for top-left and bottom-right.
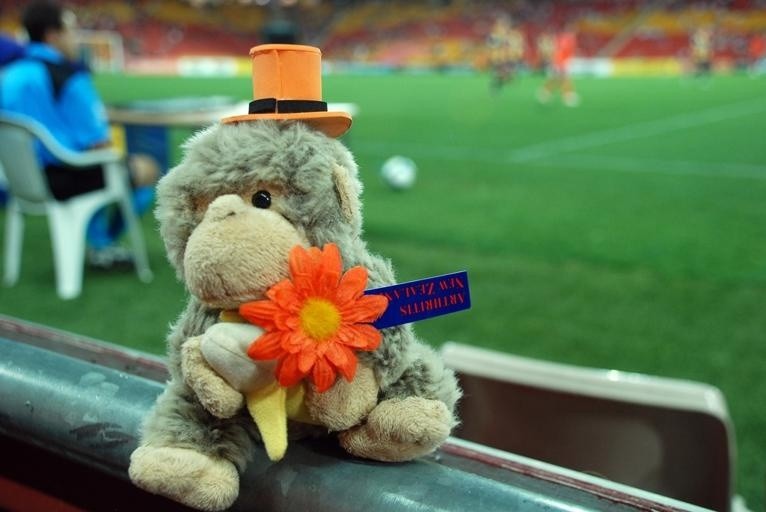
[{"x1": 385, "y1": 157, "x2": 416, "y2": 187}]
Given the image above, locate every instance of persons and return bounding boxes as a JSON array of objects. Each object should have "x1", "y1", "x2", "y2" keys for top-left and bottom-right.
[
  {"x1": 253, "y1": 1, "x2": 303, "y2": 54},
  {"x1": 0, "y1": 1, "x2": 170, "y2": 275},
  {"x1": 684, "y1": 22, "x2": 718, "y2": 87},
  {"x1": 482, "y1": 13, "x2": 583, "y2": 111}
]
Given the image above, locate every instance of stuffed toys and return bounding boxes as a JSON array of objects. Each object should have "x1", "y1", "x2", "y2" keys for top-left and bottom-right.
[{"x1": 123, "y1": 41, "x2": 465, "y2": 511}]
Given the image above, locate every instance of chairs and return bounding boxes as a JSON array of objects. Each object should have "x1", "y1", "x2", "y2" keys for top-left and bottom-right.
[
  {"x1": 435, "y1": 339, "x2": 734, "y2": 512},
  {"x1": 0, "y1": 105, "x2": 158, "y2": 303}
]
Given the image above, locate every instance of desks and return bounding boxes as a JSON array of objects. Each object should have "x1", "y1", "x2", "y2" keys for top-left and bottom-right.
[{"x1": 102, "y1": 103, "x2": 356, "y2": 248}]
